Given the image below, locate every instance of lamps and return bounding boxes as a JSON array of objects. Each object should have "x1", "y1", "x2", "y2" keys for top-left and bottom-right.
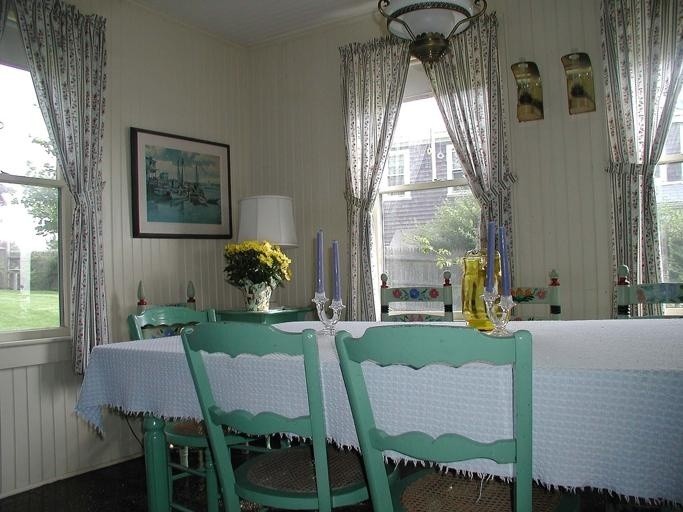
[
  {"x1": 238, "y1": 195, "x2": 300, "y2": 246},
  {"x1": 377, "y1": 0, "x2": 488, "y2": 70}
]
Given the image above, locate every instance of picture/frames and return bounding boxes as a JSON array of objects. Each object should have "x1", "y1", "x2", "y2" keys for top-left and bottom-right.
[{"x1": 131, "y1": 127, "x2": 232, "y2": 238}]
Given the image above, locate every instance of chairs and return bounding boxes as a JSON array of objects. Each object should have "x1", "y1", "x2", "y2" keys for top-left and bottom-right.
[
  {"x1": 617, "y1": 265, "x2": 683, "y2": 318},
  {"x1": 333, "y1": 324, "x2": 579, "y2": 512},
  {"x1": 137, "y1": 280, "x2": 196, "y2": 339},
  {"x1": 217, "y1": 308, "x2": 314, "y2": 325},
  {"x1": 381, "y1": 271, "x2": 453, "y2": 322},
  {"x1": 512, "y1": 270, "x2": 561, "y2": 321},
  {"x1": 179, "y1": 321, "x2": 399, "y2": 512},
  {"x1": 129, "y1": 307, "x2": 291, "y2": 510}
]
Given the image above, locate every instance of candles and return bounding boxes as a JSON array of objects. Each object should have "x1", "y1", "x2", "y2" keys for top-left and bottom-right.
[
  {"x1": 486, "y1": 219, "x2": 496, "y2": 294},
  {"x1": 332, "y1": 240, "x2": 339, "y2": 299},
  {"x1": 317, "y1": 231, "x2": 323, "y2": 293},
  {"x1": 499, "y1": 225, "x2": 511, "y2": 298}
]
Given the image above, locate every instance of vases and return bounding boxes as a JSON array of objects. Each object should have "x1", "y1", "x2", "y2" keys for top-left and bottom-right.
[{"x1": 242, "y1": 279, "x2": 273, "y2": 311}]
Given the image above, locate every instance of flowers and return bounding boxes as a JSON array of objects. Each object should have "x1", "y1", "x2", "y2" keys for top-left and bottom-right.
[{"x1": 223, "y1": 241, "x2": 292, "y2": 289}]
